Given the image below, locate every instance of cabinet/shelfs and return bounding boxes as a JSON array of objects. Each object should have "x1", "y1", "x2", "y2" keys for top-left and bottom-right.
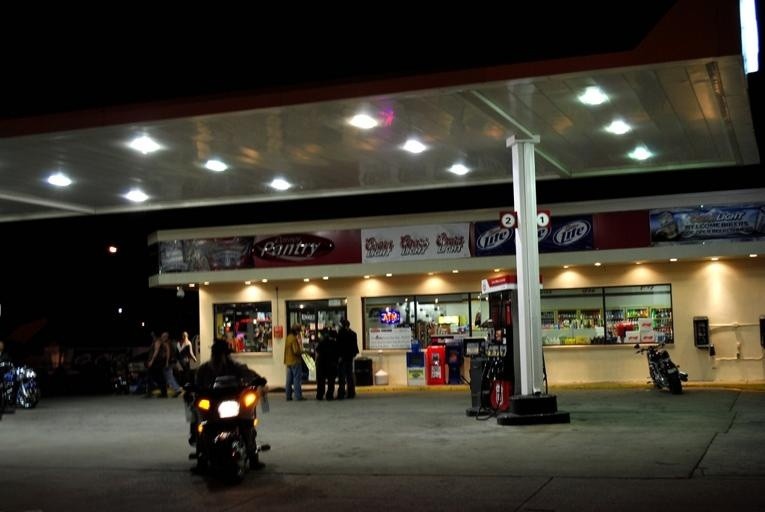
[{"x1": 541, "y1": 305, "x2": 672, "y2": 345}]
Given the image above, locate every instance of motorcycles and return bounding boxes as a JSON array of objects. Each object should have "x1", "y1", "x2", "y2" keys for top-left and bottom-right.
[
  {"x1": 0, "y1": 361, "x2": 40, "y2": 409},
  {"x1": 633, "y1": 335, "x2": 688, "y2": 396},
  {"x1": 181, "y1": 373, "x2": 271, "y2": 494}
]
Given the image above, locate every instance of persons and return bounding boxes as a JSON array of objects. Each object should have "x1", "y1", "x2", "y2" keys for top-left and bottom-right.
[
  {"x1": 140, "y1": 327, "x2": 196, "y2": 398},
  {"x1": 282, "y1": 315, "x2": 360, "y2": 400},
  {"x1": 183, "y1": 338, "x2": 270, "y2": 468}
]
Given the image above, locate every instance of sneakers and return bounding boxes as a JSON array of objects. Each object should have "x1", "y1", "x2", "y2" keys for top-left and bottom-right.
[
  {"x1": 286, "y1": 397, "x2": 293, "y2": 401},
  {"x1": 298, "y1": 396, "x2": 307, "y2": 400},
  {"x1": 316, "y1": 393, "x2": 356, "y2": 400}
]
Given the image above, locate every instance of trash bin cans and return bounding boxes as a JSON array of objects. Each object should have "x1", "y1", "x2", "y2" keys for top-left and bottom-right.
[{"x1": 355, "y1": 358, "x2": 373, "y2": 387}]
